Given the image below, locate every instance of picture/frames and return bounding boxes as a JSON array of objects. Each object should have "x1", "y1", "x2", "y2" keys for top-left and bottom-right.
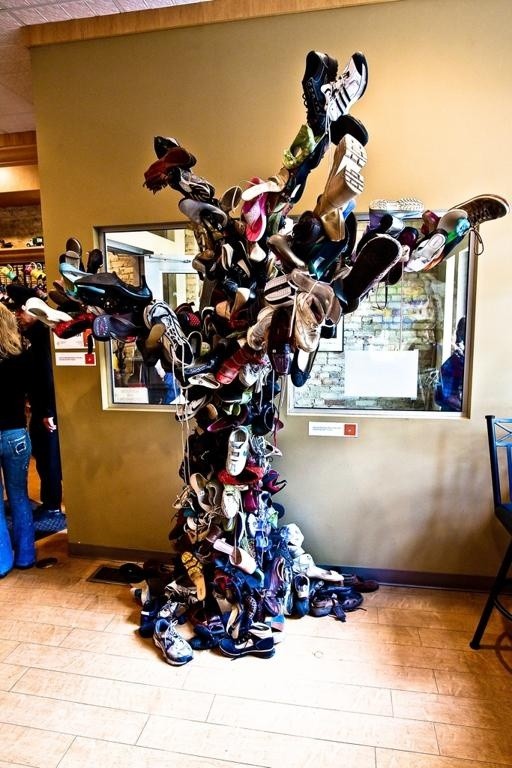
[{"x1": 317, "y1": 315, "x2": 344, "y2": 352}]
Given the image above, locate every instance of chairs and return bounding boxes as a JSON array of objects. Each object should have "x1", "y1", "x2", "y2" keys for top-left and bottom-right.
[{"x1": 469, "y1": 415, "x2": 512, "y2": 650}]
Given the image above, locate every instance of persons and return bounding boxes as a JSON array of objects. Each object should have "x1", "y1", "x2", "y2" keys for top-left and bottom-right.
[
  {"x1": 15, "y1": 305, "x2": 61, "y2": 520},
  {"x1": 0, "y1": 302, "x2": 36, "y2": 579}
]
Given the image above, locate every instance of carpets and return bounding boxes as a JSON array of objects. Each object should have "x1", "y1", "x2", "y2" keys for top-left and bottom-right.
[{"x1": 0, "y1": 497, "x2": 67, "y2": 550}]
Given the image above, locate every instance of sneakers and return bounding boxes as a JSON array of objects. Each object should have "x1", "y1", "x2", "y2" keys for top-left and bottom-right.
[
  {"x1": 120, "y1": 434, "x2": 381, "y2": 666},
  {"x1": 20, "y1": 49, "x2": 510, "y2": 433}
]
